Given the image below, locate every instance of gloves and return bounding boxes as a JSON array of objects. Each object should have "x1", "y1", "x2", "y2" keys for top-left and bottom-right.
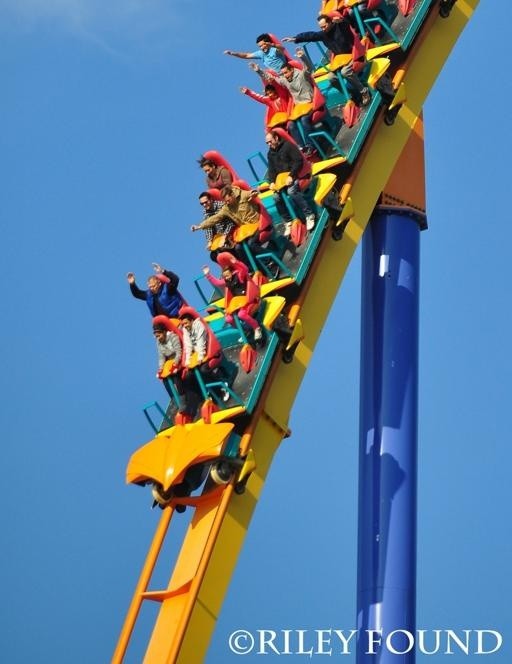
[
  {"x1": 169, "y1": 363, "x2": 179, "y2": 376},
  {"x1": 156, "y1": 370, "x2": 164, "y2": 383}
]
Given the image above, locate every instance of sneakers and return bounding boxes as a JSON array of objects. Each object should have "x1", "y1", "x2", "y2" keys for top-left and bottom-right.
[
  {"x1": 266, "y1": 262, "x2": 280, "y2": 282},
  {"x1": 306, "y1": 213, "x2": 316, "y2": 231},
  {"x1": 254, "y1": 326, "x2": 263, "y2": 341},
  {"x1": 360, "y1": 87, "x2": 370, "y2": 106},
  {"x1": 284, "y1": 220, "x2": 294, "y2": 237},
  {"x1": 238, "y1": 329, "x2": 252, "y2": 343},
  {"x1": 221, "y1": 382, "x2": 231, "y2": 402}
]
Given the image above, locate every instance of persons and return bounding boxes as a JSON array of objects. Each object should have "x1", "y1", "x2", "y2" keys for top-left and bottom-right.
[
  {"x1": 201, "y1": 256, "x2": 263, "y2": 344},
  {"x1": 125, "y1": 262, "x2": 184, "y2": 319},
  {"x1": 247, "y1": 46, "x2": 318, "y2": 159},
  {"x1": 179, "y1": 312, "x2": 231, "y2": 403},
  {"x1": 280, "y1": 14, "x2": 371, "y2": 106},
  {"x1": 264, "y1": 130, "x2": 316, "y2": 237},
  {"x1": 222, "y1": 32, "x2": 288, "y2": 73},
  {"x1": 347, "y1": 0, "x2": 378, "y2": 38},
  {"x1": 190, "y1": 187, "x2": 273, "y2": 267},
  {"x1": 196, "y1": 154, "x2": 234, "y2": 188},
  {"x1": 198, "y1": 191, "x2": 254, "y2": 278},
  {"x1": 239, "y1": 73, "x2": 291, "y2": 129},
  {"x1": 154, "y1": 325, "x2": 188, "y2": 419}
]
